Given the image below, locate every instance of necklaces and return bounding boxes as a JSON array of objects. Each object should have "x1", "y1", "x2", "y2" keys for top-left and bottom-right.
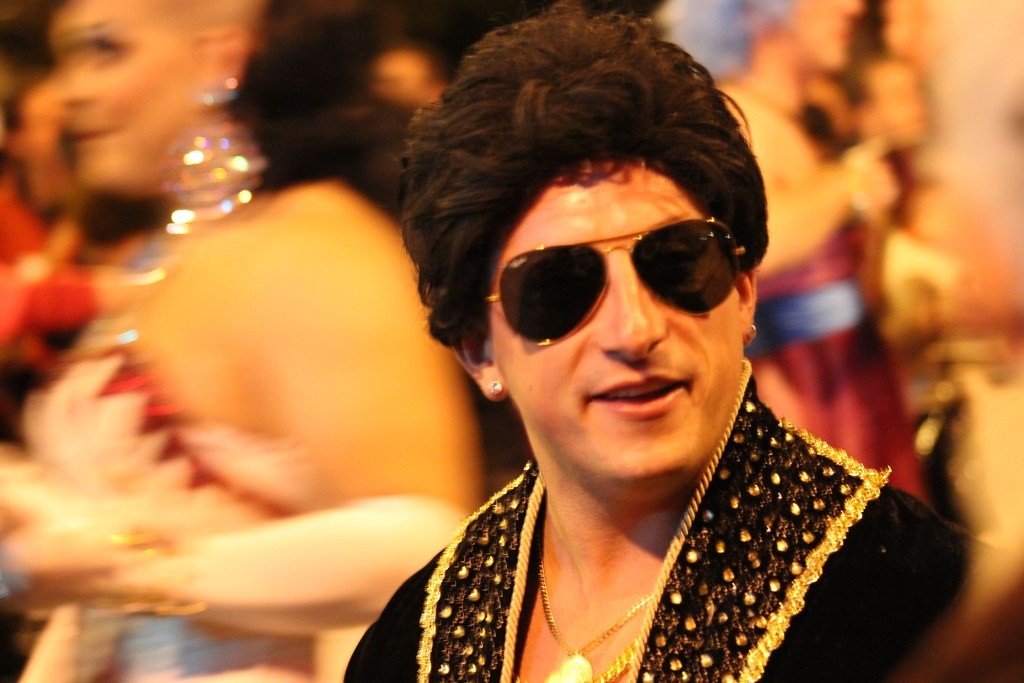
[
  {"x1": 526, "y1": 514, "x2": 654, "y2": 683},
  {"x1": 592, "y1": 634, "x2": 644, "y2": 683}
]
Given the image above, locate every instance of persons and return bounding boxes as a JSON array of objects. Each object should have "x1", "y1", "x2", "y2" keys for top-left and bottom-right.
[{"x1": 0, "y1": 0, "x2": 1023, "y2": 683}]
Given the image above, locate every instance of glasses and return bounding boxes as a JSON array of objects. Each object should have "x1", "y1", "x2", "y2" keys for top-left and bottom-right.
[{"x1": 485, "y1": 216, "x2": 747, "y2": 345}]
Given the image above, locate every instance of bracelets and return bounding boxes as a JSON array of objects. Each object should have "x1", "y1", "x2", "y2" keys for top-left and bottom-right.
[{"x1": 110, "y1": 527, "x2": 198, "y2": 621}]
